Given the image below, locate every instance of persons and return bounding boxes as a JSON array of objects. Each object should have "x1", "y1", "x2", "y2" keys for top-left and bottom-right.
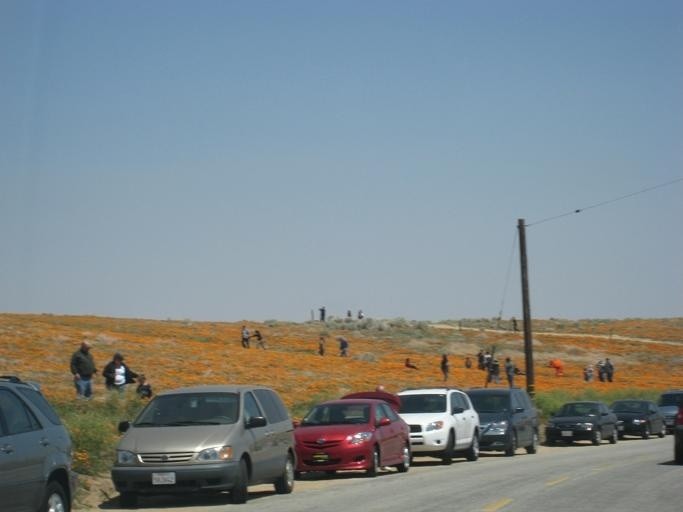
[
  {"x1": 317, "y1": 305, "x2": 325, "y2": 321},
  {"x1": 462, "y1": 346, "x2": 515, "y2": 389},
  {"x1": 337, "y1": 336, "x2": 348, "y2": 358},
  {"x1": 68, "y1": 340, "x2": 101, "y2": 400},
  {"x1": 239, "y1": 324, "x2": 250, "y2": 348},
  {"x1": 316, "y1": 336, "x2": 326, "y2": 356},
  {"x1": 404, "y1": 356, "x2": 417, "y2": 369},
  {"x1": 102, "y1": 352, "x2": 138, "y2": 393},
  {"x1": 582, "y1": 355, "x2": 614, "y2": 383},
  {"x1": 134, "y1": 372, "x2": 151, "y2": 400},
  {"x1": 440, "y1": 352, "x2": 451, "y2": 381},
  {"x1": 510, "y1": 315, "x2": 520, "y2": 331},
  {"x1": 250, "y1": 329, "x2": 267, "y2": 351},
  {"x1": 357, "y1": 309, "x2": 364, "y2": 320}
]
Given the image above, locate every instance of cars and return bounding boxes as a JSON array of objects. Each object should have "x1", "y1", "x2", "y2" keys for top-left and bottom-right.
[{"x1": 0, "y1": 375, "x2": 74, "y2": 512}]
